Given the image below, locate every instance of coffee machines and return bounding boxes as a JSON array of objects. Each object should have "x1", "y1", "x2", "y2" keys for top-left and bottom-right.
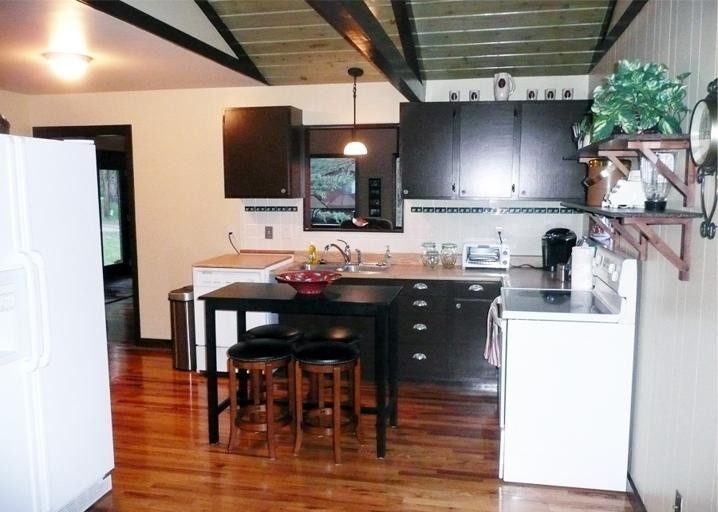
[{"x1": 541, "y1": 227, "x2": 576, "y2": 272}]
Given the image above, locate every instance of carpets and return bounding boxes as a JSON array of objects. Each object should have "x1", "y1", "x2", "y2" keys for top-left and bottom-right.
[{"x1": 104, "y1": 277, "x2": 132, "y2": 304}]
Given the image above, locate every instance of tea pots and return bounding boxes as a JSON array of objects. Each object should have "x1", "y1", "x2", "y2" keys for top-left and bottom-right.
[{"x1": 494, "y1": 72, "x2": 516, "y2": 101}]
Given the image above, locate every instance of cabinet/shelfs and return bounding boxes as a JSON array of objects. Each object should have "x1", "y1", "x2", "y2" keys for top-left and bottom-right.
[
  {"x1": 562, "y1": 134, "x2": 703, "y2": 281},
  {"x1": 517, "y1": 100, "x2": 594, "y2": 202},
  {"x1": 458, "y1": 100, "x2": 519, "y2": 200},
  {"x1": 400, "y1": 102, "x2": 457, "y2": 200},
  {"x1": 224, "y1": 106, "x2": 302, "y2": 199},
  {"x1": 398, "y1": 280, "x2": 453, "y2": 385},
  {"x1": 453, "y1": 278, "x2": 500, "y2": 392}
]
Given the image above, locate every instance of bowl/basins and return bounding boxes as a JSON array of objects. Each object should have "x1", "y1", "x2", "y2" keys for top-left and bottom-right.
[{"x1": 273, "y1": 270, "x2": 341, "y2": 296}]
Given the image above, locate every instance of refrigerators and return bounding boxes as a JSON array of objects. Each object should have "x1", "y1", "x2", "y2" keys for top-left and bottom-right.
[{"x1": 0, "y1": 133, "x2": 116, "y2": 512}]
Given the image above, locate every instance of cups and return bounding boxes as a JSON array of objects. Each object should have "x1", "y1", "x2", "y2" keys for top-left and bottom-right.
[
  {"x1": 448, "y1": 90, "x2": 460, "y2": 102},
  {"x1": 526, "y1": 89, "x2": 538, "y2": 100},
  {"x1": 562, "y1": 88, "x2": 574, "y2": 100},
  {"x1": 543, "y1": 88, "x2": 556, "y2": 101},
  {"x1": 469, "y1": 90, "x2": 479, "y2": 101}
]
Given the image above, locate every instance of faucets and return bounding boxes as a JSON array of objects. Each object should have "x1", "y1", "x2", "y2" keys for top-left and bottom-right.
[{"x1": 325, "y1": 239, "x2": 352, "y2": 264}]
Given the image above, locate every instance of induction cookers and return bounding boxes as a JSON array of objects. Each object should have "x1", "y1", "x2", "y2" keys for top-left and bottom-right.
[{"x1": 504, "y1": 286, "x2": 613, "y2": 318}]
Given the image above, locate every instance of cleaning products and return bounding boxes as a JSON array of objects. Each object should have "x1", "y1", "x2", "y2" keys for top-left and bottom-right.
[{"x1": 309, "y1": 242, "x2": 318, "y2": 264}]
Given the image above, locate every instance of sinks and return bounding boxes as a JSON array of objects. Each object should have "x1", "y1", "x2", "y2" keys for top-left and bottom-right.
[
  {"x1": 298, "y1": 264, "x2": 339, "y2": 272},
  {"x1": 342, "y1": 265, "x2": 388, "y2": 273}
]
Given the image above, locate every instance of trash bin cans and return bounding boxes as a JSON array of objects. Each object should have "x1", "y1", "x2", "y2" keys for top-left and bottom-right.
[{"x1": 169, "y1": 285, "x2": 196, "y2": 371}]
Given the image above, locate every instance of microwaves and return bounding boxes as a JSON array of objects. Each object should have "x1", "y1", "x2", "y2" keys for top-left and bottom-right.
[{"x1": 462, "y1": 243, "x2": 510, "y2": 271}]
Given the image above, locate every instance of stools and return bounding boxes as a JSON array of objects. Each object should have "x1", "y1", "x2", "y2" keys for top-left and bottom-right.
[{"x1": 226, "y1": 324, "x2": 365, "y2": 466}]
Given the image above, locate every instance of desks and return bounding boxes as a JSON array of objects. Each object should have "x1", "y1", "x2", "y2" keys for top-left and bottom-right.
[{"x1": 197, "y1": 282, "x2": 403, "y2": 459}]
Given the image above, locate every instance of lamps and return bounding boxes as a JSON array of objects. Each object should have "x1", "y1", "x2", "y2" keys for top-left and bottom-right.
[{"x1": 344, "y1": 67, "x2": 367, "y2": 157}]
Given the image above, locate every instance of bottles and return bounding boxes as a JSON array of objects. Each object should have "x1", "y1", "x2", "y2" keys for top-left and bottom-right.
[
  {"x1": 307, "y1": 242, "x2": 318, "y2": 265},
  {"x1": 638, "y1": 153, "x2": 675, "y2": 212},
  {"x1": 556, "y1": 263, "x2": 569, "y2": 281},
  {"x1": 441, "y1": 243, "x2": 457, "y2": 269},
  {"x1": 421, "y1": 241, "x2": 440, "y2": 269}
]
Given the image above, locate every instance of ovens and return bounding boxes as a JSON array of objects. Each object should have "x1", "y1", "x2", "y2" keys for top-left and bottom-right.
[{"x1": 489, "y1": 296, "x2": 637, "y2": 495}]
